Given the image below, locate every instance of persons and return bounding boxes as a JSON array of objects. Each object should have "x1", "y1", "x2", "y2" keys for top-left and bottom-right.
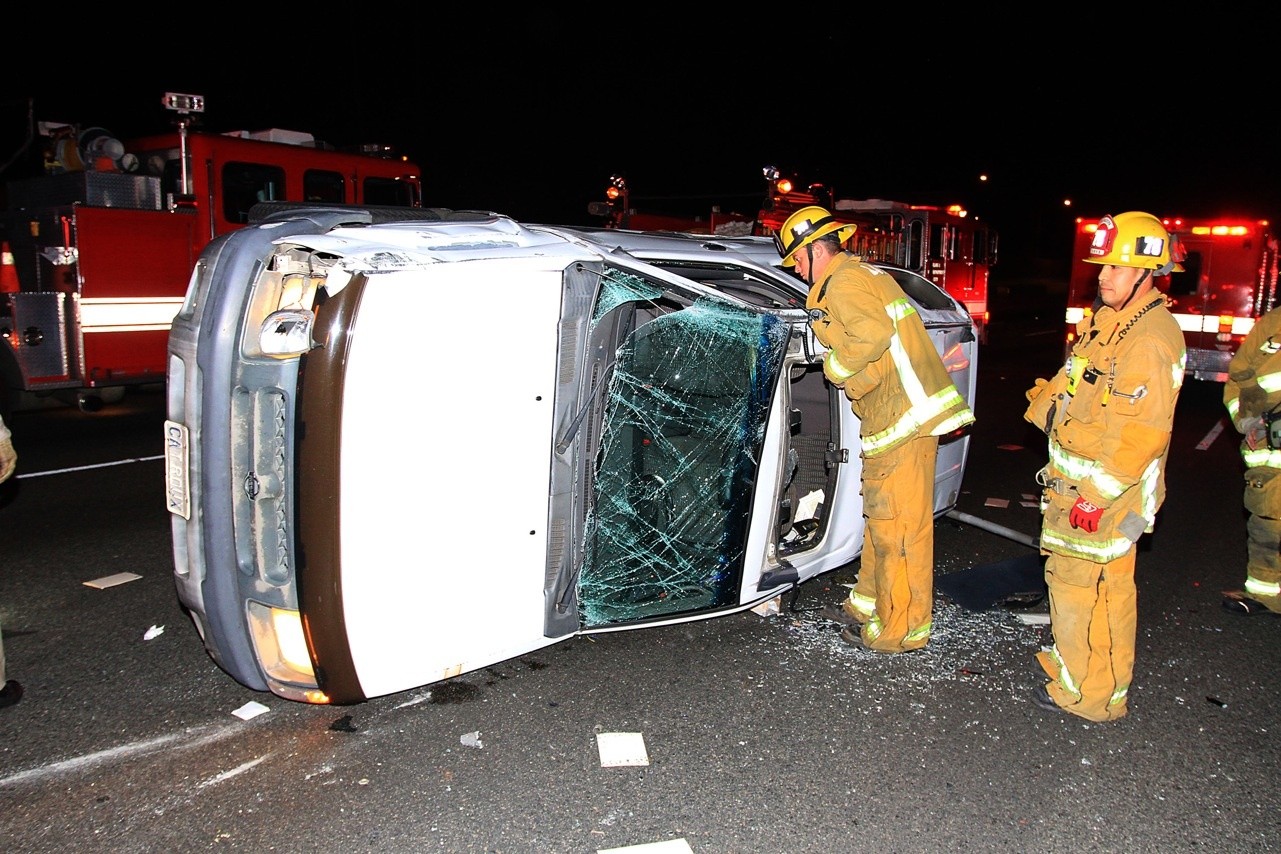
[
  {"x1": 1220, "y1": 305, "x2": 1281, "y2": 616},
  {"x1": 1024, "y1": 211, "x2": 1186, "y2": 721},
  {"x1": 772, "y1": 206, "x2": 976, "y2": 653},
  {"x1": 0, "y1": 415, "x2": 23, "y2": 706}
]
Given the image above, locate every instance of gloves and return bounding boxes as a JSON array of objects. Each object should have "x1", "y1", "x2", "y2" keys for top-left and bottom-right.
[{"x1": 1069, "y1": 496, "x2": 1104, "y2": 533}]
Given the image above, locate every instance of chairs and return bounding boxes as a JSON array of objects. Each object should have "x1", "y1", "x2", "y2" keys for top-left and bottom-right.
[
  {"x1": 643, "y1": 298, "x2": 771, "y2": 401},
  {"x1": 643, "y1": 442, "x2": 758, "y2": 551}
]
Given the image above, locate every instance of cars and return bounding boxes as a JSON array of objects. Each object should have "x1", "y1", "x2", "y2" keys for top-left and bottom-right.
[{"x1": 165, "y1": 206, "x2": 979, "y2": 707}]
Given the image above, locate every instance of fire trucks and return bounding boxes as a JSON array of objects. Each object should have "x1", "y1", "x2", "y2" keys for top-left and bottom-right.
[
  {"x1": 0, "y1": 91, "x2": 422, "y2": 423},
  {"x1": 1062, "y1": 217, "x2": 1281, "y2": 422},
  {"x1": 585, "y1": 165, "x2": 998, "y2": 342}
]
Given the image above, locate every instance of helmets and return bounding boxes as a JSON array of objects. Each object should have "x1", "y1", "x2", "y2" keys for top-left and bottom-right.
[
  {"x1": 771, "y1": 206, "x2": 857, "y2": 268},
  {"x1": 1082, "y1": 211, "x2": 1186, "y2": 273}
]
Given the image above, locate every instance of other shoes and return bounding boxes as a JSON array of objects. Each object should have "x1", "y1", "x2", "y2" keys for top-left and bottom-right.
[
  {"x1": 841, "y1": 625, "x2": 865, "y2": 644},
  {"x1": 1031, "y1": 654, "x2": 1052, "y2": 682},
  {"x1": 1030, "y1": 685, "x2": 1064, "y2": 711},
  {"x1": 1222, "y1": 597, "x2": 1274, "y2": 615},
  {"x1": 824, "y1": 604, "x2": 860, "y2": 624},
  {"x1": 0, "y1": 680, "x2": 22, "y2": 709}
]
